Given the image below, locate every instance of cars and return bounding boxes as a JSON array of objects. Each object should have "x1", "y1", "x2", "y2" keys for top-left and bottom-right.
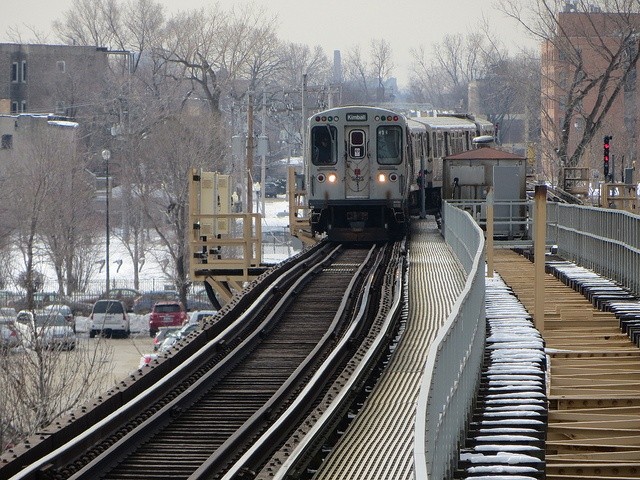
[
  {"x1": 0, "y1": 307, "x2": 17, "y2": 325},
  {"x1": 45, "y1": 305, "x2": 76, "y2": 333},
  {"x1": 0, "y1": 324, "x2": 22, "y2": 350},
  {"x1": 8, "y1": 292, "x2": 93, "y2": 316},
  {"x1": 131, "y1": 290, "x2": 210, "y2": 313},
  {"x1": 15, "y1": 310, "x2": 77, "y2": 350},
  {"x1": 100, "y1": 289, "x2": 142, "y2": 312},
  {"x1": 188, "y1": 310, "x2": 217, "y2": 324},
  {"x1": 153, "y1": 326, "x2": 180, "y2": 351},
  {"x1": 265, "y1": 182, "x2": 277, "y2": 198}
]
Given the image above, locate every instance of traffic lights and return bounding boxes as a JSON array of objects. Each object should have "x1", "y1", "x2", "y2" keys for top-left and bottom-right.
[{"x1": 604, "y1": 136, "x2": 612, "y2": 176}]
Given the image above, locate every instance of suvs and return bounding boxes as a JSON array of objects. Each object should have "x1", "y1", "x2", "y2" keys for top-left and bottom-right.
[
  {"x1": 89, "y1": 299, "x2": 130, "y2": 339},
  {"x1": 150, "y1": 301, "x2": 187, "y2": 337}
]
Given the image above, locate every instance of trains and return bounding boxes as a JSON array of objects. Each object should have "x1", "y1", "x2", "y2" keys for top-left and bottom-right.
[{"x1": 304, "y1": 105, "x2": 495, "y2": 242}]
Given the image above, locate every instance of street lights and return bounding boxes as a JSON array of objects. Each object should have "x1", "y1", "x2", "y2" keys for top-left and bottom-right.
[
  {"x1": 96, "y1": 48, "x2": 130, "y2": 241},
  {"x1": 284, "y1": 90, "x2": 307, "y2": 165},
  {"x1": 232, "y1": 192, "x2": 239, "y2": 259},
  {"x1": 255, "y1": 182, "x2": 260, "y2": 213},
  {"x1": 101, "y1": 149, "x2": 111, "y2": 299}
]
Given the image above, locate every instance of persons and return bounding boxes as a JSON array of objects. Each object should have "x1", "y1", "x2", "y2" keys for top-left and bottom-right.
[
  {"x1": 254, "y1": 181, "x2": 260, "y2": 197},
  {"x1": 231, "y1": 192, "x2": 239, "y2": 207}
]
